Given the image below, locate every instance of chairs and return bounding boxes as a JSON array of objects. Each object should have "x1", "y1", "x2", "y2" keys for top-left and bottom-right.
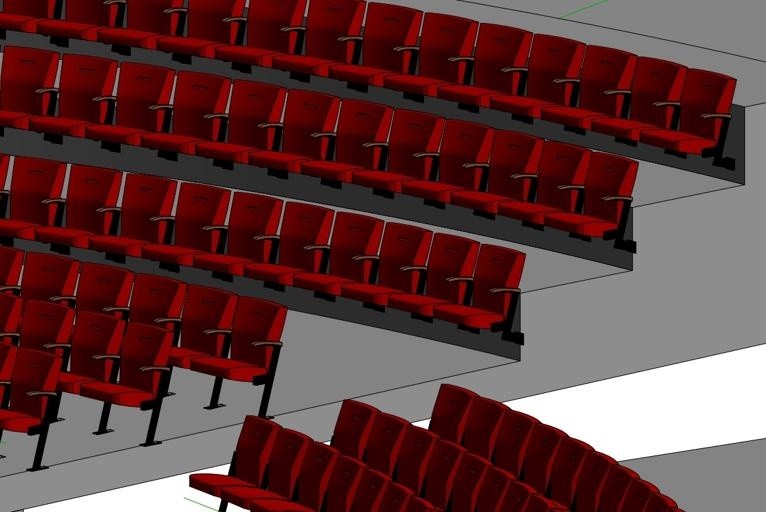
[{"x1": 0, "y1": 0, "x2": 739, "y2": 511}]
